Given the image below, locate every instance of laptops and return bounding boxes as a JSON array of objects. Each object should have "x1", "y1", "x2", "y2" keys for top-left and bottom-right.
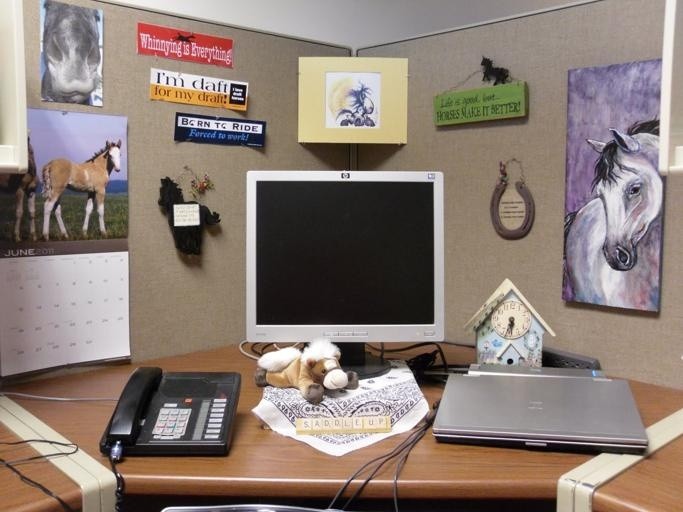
[{"x1": 432, "y1": 362, "x2": 649, "y2": 453}]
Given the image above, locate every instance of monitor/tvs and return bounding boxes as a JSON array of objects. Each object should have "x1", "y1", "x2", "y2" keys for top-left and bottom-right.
[{"x1": 245, "y1": 170, "x2": 445, "y2": 381}]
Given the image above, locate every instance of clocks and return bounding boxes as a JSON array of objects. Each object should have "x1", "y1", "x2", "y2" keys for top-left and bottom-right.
[{"x1": 462, "y1": 278, "x2": 556, "y2": 367}]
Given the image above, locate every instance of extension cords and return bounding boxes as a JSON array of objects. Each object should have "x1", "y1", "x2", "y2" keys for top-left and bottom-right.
[{"x1": 542, "y1": 347, "x2": 600, "y2": 370}]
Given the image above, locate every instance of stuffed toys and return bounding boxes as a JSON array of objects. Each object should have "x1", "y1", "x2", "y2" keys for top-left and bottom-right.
[{"x1": 255, "y1": 339, "x2": 359, "y2": 403}]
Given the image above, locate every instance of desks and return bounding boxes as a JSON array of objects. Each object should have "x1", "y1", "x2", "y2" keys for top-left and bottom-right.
[
  {"x1": 575, "y1": 409, "x2": 683, "y2": 511},
  {"x1": 0, "y1": 403, "x2": 101, "y2": 512},
  {"x1": 0, "y1": 343, "x2": 682, "y2": 511}
]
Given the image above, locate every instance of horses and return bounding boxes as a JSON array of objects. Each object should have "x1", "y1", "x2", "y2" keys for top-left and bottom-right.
[
  {"x1": 0, "y1": 136, "x2": 38, "y2": 243},
  {"x1": 40, "y1": 138, "x2": 122, "y2": 242}
]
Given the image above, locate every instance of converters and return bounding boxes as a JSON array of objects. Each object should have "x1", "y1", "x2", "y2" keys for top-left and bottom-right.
[{"x1": 413, "y1": 352, "x2": 432, "y2": 377}]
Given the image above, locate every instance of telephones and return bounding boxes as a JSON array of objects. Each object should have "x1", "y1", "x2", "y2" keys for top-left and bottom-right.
[{"x1": 100, "y1": 366, "x2": 241, "y2": 463}]
